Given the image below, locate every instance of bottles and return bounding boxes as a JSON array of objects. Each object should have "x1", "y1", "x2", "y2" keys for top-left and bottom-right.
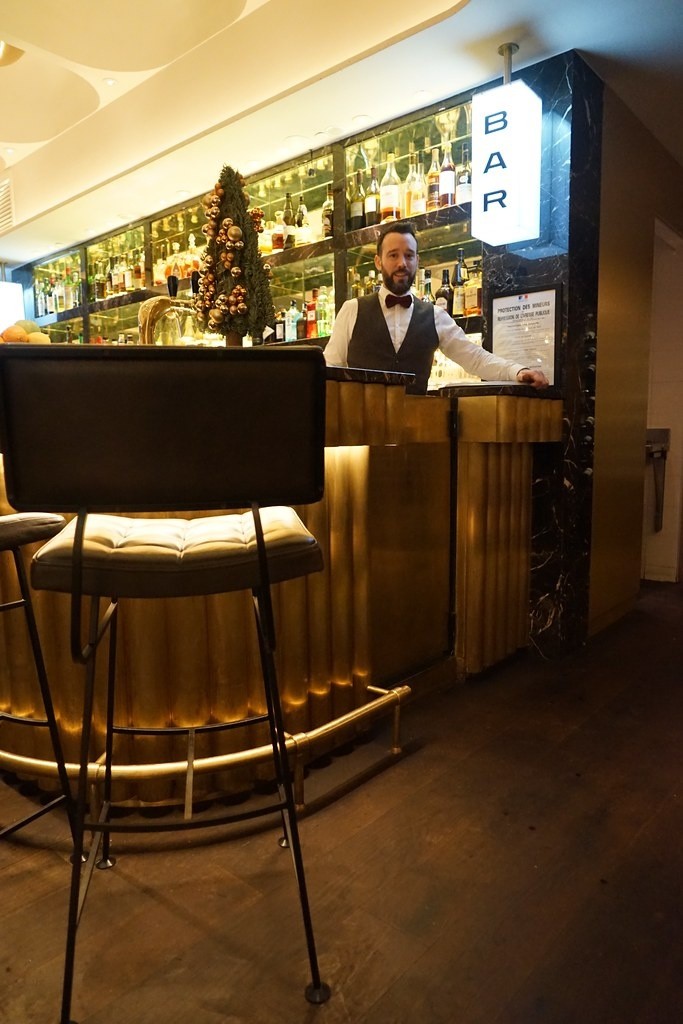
[
  {"x1": 257, "y1": 183, "x2": 334, "y2": 257},
  {"x1": 349, "y1": 248, "x2": 481, "y2": 317},
  {"x1": 154, "y1": 233, "x2": 203, "y2": 284},
  {"x1": 38, "y1": 255, "x2": 145, "y2": 317},
  {"x1": 349, "y1": 142, "x2": 471, "y2": 230},
  {"x1": 66, "y1": 324, "x2": 134, "y2": 344},
  {"x1": 264, "y1": 285, "x2": 335, "y2": 344}
]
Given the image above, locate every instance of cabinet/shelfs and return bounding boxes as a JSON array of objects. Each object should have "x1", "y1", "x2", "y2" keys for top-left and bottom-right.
[{"x1": 11, "y1": 84, "x2": 487, "y2": 346}]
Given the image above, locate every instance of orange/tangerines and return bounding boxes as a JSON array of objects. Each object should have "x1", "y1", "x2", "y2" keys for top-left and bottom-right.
[
  {"x1": 15, "y1": 320, "x2": 41, "y2": 334},
  {"x1": 3, "y1": 325, "x2": 27, "y2": 342},
  {"x1": 28, "y1": 332, "x2": 50, "y2": 343}
]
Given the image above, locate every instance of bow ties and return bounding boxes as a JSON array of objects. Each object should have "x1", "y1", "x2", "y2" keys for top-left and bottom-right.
[{"x1": 385, "y1": 294, "x2": 411, "y2": 309}]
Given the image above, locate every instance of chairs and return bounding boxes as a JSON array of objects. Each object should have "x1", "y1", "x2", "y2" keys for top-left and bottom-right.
[{"x1": 0, "y1": 343, "x2": 329, "y2": 1024}]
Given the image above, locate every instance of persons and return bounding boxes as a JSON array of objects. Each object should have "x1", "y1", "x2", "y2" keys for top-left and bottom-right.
[{"x1": 321, "y1": 225, "x2": 556, "y2": 391}]
